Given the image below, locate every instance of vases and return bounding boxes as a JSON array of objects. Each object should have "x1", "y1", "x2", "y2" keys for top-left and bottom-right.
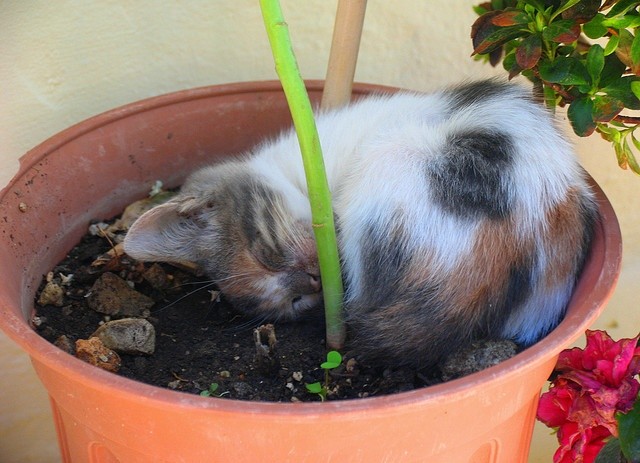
[{"x1": 0, "y1": 78, "x2": 624, "y2": 461}]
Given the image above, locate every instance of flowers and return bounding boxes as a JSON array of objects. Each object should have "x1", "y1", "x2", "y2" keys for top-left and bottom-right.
[{"x1": 537, "y1": 323, "x2": 639, "y2": 461}]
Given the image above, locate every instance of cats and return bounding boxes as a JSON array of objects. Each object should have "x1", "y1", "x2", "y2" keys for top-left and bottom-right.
[{"x1": 123, "y1": 72, "x2": 602, "y2": 371}]
